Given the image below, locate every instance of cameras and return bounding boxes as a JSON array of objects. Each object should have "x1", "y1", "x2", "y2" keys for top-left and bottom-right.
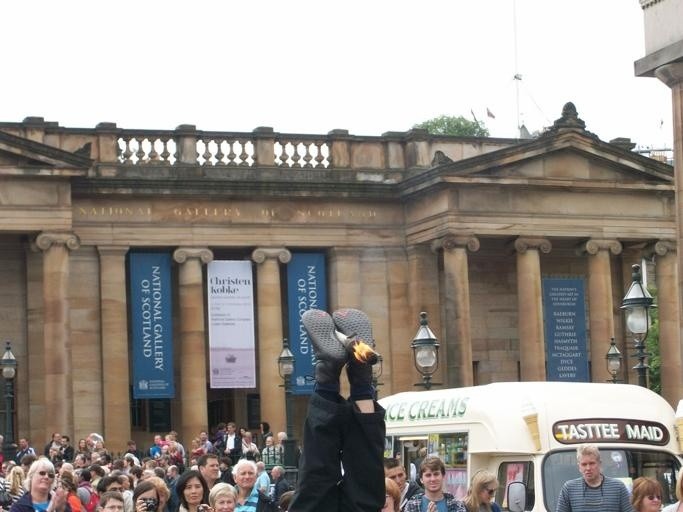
[
  {"x1": 141, "y1": 496, "x2": 157, "y2": 512},
  {"x1": 196, "y1": 505, "x2": 206, "y2": 512}
]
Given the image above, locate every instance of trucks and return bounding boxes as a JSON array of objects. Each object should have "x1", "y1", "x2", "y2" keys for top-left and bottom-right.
[{"x1": 369, "y1": 378, "x2": 682, "y2": 512}]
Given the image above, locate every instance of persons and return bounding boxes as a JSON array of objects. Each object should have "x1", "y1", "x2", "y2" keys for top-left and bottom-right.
[
  {"x1": 556, "y1": 444, "x2": 683, "y2": 512},
  {"x1": 383, "y1": 455, "x2": 502, "y2": 512},
  {"x1": 288, "y1": 307, "x2": 385, "y2": 512},
  {"x1": 1, "y1": 421, "x2": 302, "y2": 512}
]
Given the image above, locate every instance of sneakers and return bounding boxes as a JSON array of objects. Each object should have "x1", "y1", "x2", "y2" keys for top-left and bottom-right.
[
  {"x1": 331, "y1": 307, "x2": 373, "y2": 372},
  {"x1": 300, "y1": 307, "x2": 349, "y2": 365}
]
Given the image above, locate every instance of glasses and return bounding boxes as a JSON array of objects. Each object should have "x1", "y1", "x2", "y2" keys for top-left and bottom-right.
[{"x1": 34, "y1": 470, "x2": 55, "y2": 478}]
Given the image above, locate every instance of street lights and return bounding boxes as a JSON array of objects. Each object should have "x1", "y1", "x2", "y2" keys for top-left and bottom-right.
[
  {"x1": 0, "y1": 337, "x2": 22, "y2": 466},
  {"x1": 408, "y1": 308, "x2": 445, "y2": 391},
  {"x1": 372, "y1": 338, "x2": 385, "y2": 404},
  {"x1": 619, "y1": 261, "x2": 657, "y2": 388},
  {"x1": 274, "y1": 335, "x2": 300, "y2": 469},
  {"x1": 601, "y1": 336, "x2": 625, "y2": 386}
]
